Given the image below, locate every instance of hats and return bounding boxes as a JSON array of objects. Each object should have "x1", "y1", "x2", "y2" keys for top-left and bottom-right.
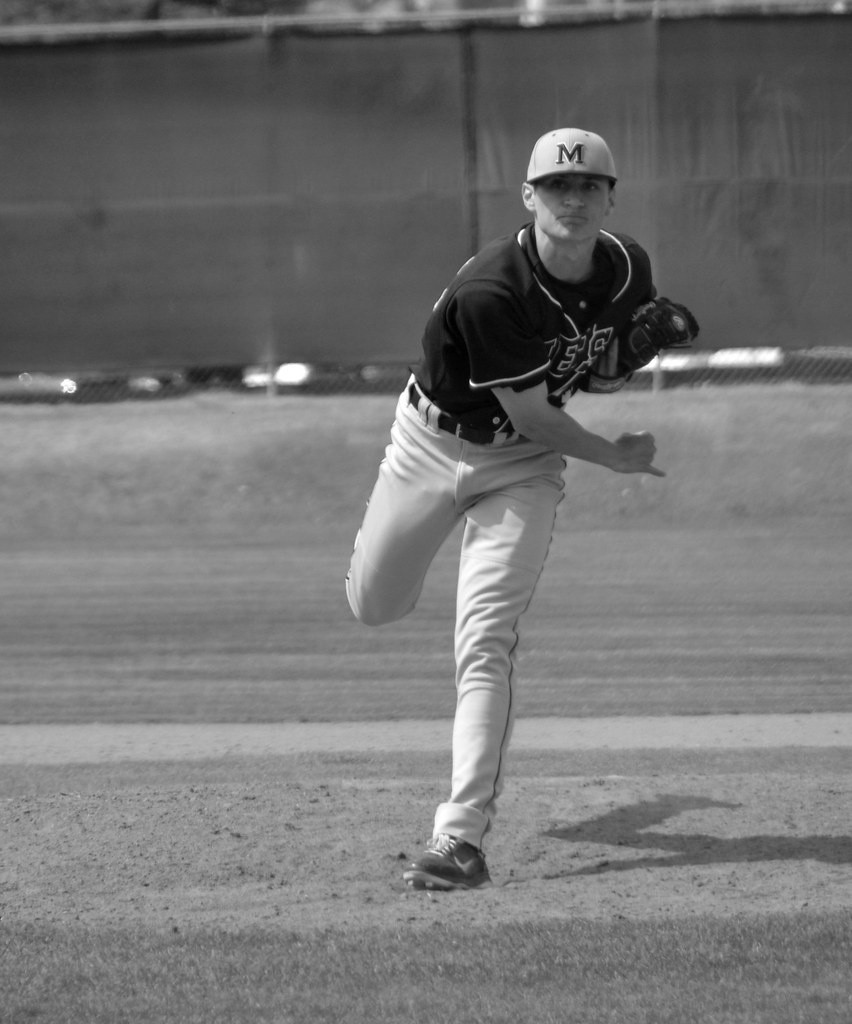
[{"x1": 526, "y1": 128, "x2": 617, "y2": 186}]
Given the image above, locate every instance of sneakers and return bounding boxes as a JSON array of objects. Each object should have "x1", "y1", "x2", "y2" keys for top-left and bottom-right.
[{"x1": 403, "y1": 836, "x2": 492, "y2": 893}]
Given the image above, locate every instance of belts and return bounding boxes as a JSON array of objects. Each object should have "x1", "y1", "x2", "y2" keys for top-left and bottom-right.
[{"x1": 408, "y1": 383, "x2": 523, "y2": 445}]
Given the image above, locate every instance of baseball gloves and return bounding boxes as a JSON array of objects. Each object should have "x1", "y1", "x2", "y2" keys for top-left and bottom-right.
[{"x1": 583, "y1": 295, "x2": 701, "y2": 394}]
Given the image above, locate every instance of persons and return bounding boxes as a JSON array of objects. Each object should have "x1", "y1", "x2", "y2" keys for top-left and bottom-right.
[{"x1": 346, "y1": 126, "x2": 665, "y2": 891}]
